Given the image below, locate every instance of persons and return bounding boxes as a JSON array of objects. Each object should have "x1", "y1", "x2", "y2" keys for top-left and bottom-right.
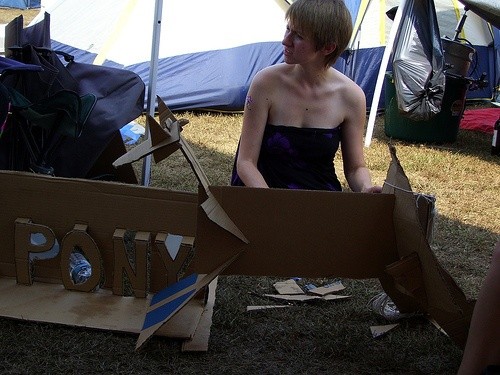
[{"x1": 231, "y1": 0, "x2": 382, "y2": 194}]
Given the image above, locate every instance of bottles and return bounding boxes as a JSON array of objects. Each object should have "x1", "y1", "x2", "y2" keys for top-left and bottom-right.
[
  {"x1": 491, "y1": 118, "x2": 500, "y2": 156},
  {"x1": 69, "y1": 252, "x2": 99, "y2": 290}
]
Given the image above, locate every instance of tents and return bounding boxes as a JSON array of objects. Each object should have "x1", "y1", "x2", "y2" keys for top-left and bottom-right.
[{"x1": 24, "y1": 0, "x2": 499, "y2": 118}]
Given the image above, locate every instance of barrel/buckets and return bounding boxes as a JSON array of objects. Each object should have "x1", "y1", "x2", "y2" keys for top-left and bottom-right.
[{"x1": 441, "y1": 36, "x2": 476, "y2": 77}]
